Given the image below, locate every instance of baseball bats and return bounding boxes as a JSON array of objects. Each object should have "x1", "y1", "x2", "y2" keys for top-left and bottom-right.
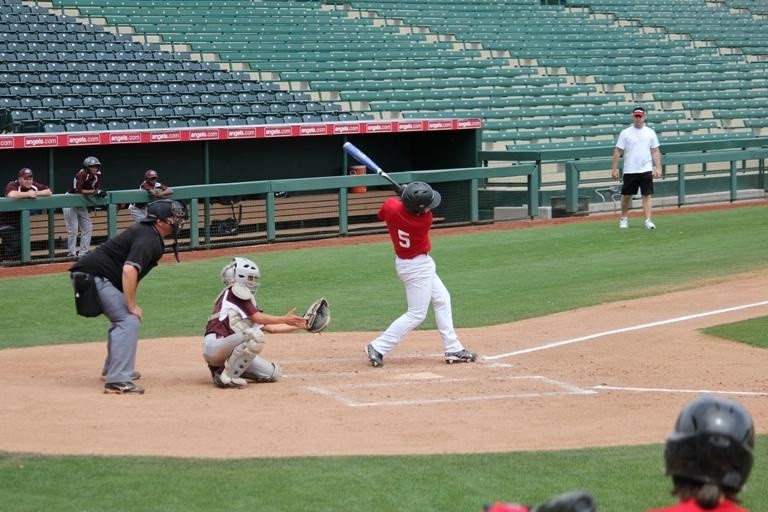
[{"x1": 342, "y1": 142, "x2": 400, "y2": 195}]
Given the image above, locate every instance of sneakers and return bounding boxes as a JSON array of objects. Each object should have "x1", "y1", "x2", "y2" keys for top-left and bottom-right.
[
  {"x1": 619, "y1": 217, "x2": 628, "y2": 228},
  {"x1": 100, "y1": 371, "x2": 145, "y2": 395},
  {"x1": 645, "y1": 219, "x2": 656, "y2": 230},
  {"x1": 365, "y1": 344, "x2": 383, "y2": 366},
  {"x1": 445, "y1": 350, "x2": 476, "y2": 364},
  {"x1": 216, "y1": 372, "x2": 246, "y2": 387}
]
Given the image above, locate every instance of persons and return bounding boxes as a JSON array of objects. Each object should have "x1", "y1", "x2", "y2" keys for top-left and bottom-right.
[
  {"x1": 643, "y1": 394, "x2": 755, "y2": 512},
  {"x1": 610, "y1": 107, "x2": 662, "y2": 230},
  {"x1": 127, "y1": 169, "x2": 174, "y2": 222},
  {"x1": 68, "y1": 199, "x2": 191, "y2": 395},
  {"x1": 0, "y1": 168, "x2": 53, "y2": 261},
  {"x1": 204, "y1": 256, "x2": 308, "y2": 389},
  {"x1": 361, "y1": 182, "x2": 479, "y2": 367},
  {"x1": 62, "y1": 157, "x2": 107, "y2": 258}
]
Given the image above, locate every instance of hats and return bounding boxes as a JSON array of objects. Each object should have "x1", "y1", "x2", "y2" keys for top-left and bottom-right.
[
  {"x1": 19, "y1": 169, "x2": 33, "y2": 178},
  {"x1": 144, "y1": 170, "x2": 157, "y2": 179},
  {"x1": 633, "y1": 108, "x2": 644, "y2": 117}
]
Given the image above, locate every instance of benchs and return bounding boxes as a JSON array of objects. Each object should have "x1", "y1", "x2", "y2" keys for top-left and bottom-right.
[{"x1": 23, "y1": 188, "x2": 447, "y2": 254}]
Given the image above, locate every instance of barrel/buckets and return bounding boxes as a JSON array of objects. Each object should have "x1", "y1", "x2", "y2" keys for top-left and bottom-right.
[{"x1": 348, "y1": 165, "x2": 367, "y2": 193}]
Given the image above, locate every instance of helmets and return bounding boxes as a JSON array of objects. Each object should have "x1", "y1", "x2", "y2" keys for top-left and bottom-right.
[
  {"x1": 139, "y1": 199, "x2": 186, "y2": 262},
  {"x1": 401, "y1": 181, "x2": 441, "y2": 212},
  {"x1": 221, "y1": 257, "x2": 261, "y2": 299},
  {"x1": 84, "y1": 157, "x2": 101, "y2": 171},
  {"x1": 665, "y1": 396, "x2": 754, "y2": 490}
]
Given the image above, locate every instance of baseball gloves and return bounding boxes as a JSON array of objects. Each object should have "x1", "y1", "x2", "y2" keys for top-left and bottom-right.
[{"x1": 303, "y1": 299, "x2": 330, "y2": 334}]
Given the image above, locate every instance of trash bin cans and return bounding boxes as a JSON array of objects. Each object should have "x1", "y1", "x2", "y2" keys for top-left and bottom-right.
[{"x1": 549, "y1": 195, "x2": 591, "y2": 219}]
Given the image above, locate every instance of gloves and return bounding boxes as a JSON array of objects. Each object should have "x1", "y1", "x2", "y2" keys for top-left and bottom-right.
[{"x1": 94, "y1": 190, "x2": 105, "y2": 197}]
